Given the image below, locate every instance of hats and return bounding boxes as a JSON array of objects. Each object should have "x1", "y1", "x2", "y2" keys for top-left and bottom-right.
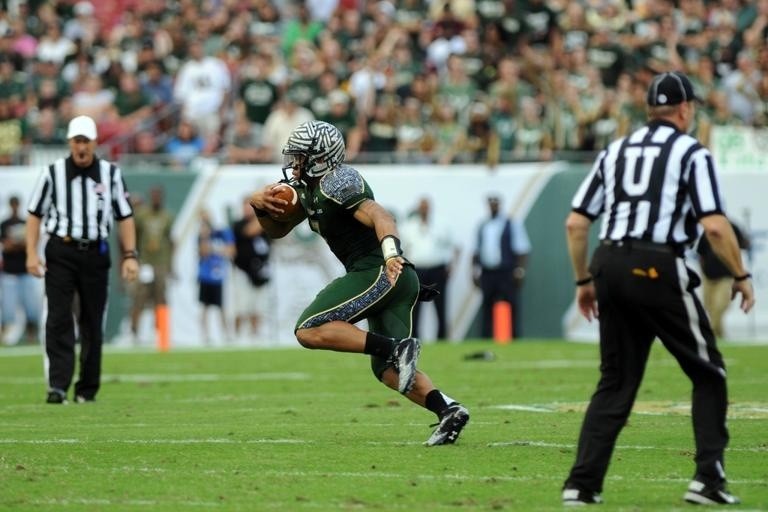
[
  {"x1": 647, "y1": 72, "x2": 705, "y2": 110},
  {"x1": 66, "y1": 114, "x2": 98, "y2": 142}
]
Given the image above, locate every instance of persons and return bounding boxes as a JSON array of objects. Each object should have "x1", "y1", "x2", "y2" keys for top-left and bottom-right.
[
  {"x1": 247, "y1": 123, "x2": 470, "y2": 446},
  {"x1": 466, "y1": 189, "x2": 533, "y2": 340},
  {"x1": 552, "y1": 67, "x2": 761, "y2": 509},
  {"x1": 691, "y1": 208, "x2": 754, "y2": 344},
  {"x1": 22, "y1": 114, "x2": 142, "y2": 407},
  {"x1": 394, "y1": 192, "x2": 453, "y2": 342},
  {"x1": 1, "y1": 194, "x2": 46, "y2": 347},
  {"x1": 119, "y1": 182, "x2": 279, "y2": 350},
  {"x1": 1, "y1": 1, "x2": 768, "y2": 166}
]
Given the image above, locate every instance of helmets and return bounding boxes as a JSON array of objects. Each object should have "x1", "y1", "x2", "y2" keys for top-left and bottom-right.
[{"x1": 281, "y1": 120, "x2": 346, "y2": 188}]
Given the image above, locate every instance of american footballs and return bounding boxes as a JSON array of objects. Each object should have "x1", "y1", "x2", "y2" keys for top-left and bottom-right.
[{"x1": 269, "y1": 183, "x2": 302, "y2": 223}]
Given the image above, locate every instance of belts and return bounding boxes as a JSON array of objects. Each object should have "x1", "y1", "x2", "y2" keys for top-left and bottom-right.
[
  {"x1": 599, "y1": 238, "x2": 678, "y2": 256},
  {"x1": 50, "y1": 234, "x2": 103, "y2": 251}
]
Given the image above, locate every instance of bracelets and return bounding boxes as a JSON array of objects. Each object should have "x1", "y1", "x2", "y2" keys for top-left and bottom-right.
[
  {"x1": 735, "y1": 273, "x2": 751, "y2": 282},
  {"x1": 576, "y1": 276, "x2": 592, "y2": 286},
  {"x1": 380, "y1": 234, "x2": 403, "y2": 263}
]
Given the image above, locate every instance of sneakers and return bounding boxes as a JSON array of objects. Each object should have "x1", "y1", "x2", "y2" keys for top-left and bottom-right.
[
  {"x1": 390, "y1": 337, "x2": 421, "y2": 394},
  {"x1": 563, "y1": 486, "x2": 603, "y2": 506},
  {"x1": 74, "y1": 394, "x2": 94, "y2": 402},
  {"x1": 47, "y1": 390, "x2": 67, "y2": 404},
  {"x1": 422, "y1": 401, "x2": 469, "y2": 449},
  {"x1": 682, "y1": 477, "x2": 739, "y2": 506}
]
